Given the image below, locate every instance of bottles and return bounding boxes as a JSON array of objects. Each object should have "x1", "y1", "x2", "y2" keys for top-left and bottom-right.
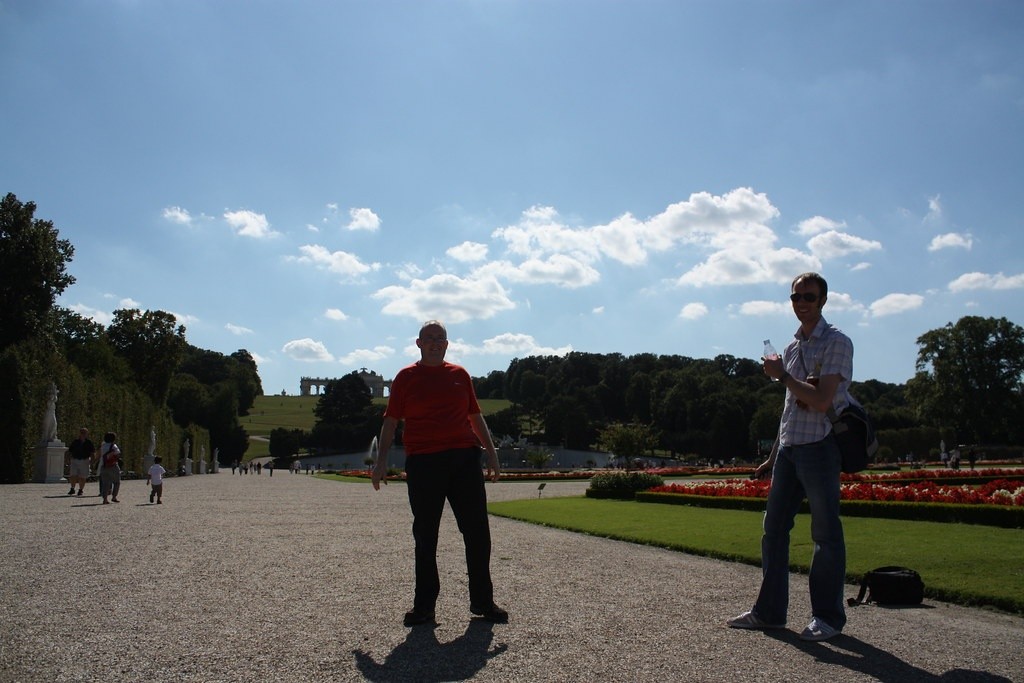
[{"x1": 763, "y1": 338, "x2": 780, "y2": 382}]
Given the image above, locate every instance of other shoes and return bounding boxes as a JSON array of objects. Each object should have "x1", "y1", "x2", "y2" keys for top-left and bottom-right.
[
  {"x1": 103, "y1": 501, "x2": 109, "y2": 504},
  {"x1": 405, "y1": 606, "x2": 435, "y2": 623},
  {"x1": 150, "y1": 495, "x2": 153, "y2": 503},
  {"x1": 99, "y1": 493, "x2": 103, "y2": 496},
  {"x1": 108, "y1": 491, "x2": 112, "y2": 495},
  {"x1": 112, "y1": 499, "x2": 120, "y2": 502},
  {"x1": 77, "y1": 489, "x2": 83, "y2": 495},
  {"x1": 470, "y1": 600, "x2": 508, "y2": 620},
  {"x1": 68, "y1": 488, "x2": 75, "y2": 494},
  {"x1": 157, "y1": 501, "x2": 162, "y2": 504}
]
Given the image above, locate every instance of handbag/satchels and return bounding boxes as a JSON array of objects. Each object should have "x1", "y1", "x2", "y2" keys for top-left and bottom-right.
[
  {"x1": 847, "y1": 566, "x2": 925, "y2": 607},
  {"x1": 103, "y1": 444, "x2": 117, "y2": 469},
  {"x1": 835, "y1": 405, "x2": 878, "y2": 474}
]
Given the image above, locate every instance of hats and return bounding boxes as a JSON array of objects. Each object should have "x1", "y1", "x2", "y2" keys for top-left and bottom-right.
[{"x1": 80, "y1": 428, "x2": 88, "y2": 432}]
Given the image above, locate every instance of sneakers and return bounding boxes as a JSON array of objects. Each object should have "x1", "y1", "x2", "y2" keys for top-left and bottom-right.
[
  {"x1": 726, "y1": 610, "x2": 785, "y2": 628},
  {"x1": 800, "y1": 616, "x2": 841, "y2": 641}
]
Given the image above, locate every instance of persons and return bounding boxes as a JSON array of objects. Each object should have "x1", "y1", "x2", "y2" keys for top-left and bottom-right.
[
  {"x1": 952, "y1": 447, "x2": 961, "y2": 468},
  {"x1": 371, "y1": 324, "x2": 509, "y2": 623},
  {"x1": 728, "y1": 274, "x2": 854, "y2": 640},
  {"x1": 43, "y1": 392, "x2": 57, "y2": 441},
  {"x1": 183, "y1": 438, "x2": 190, "y2": 458},
  {"x1": 214, "y1": 447, "x2": 218, "y2": 461},
  {"x1": 148, "y1": 425, "x2": 156, "y2": 455},
  {"x1": 199, "y1": 445, "x2": 205, "y2": 460},
  {"x1": 147, "y1": 456, "x2": 166, "y2": 504},
  {"x1": 269, "y1": 461, "x2": 274, "y2": 476},
  {"x1": 65, "y1": 428, "x2": 121, "y2": 504},
  {"x1": 909, "y1": 452, "x2": 913, "y2": 469},
  {"x1": 231, "y1": 461, "x2": 261, "y2": 475},
  {"x1": 306, "y1": 464, "x2": 315, "y2": 475},
  {"x1": 942, "y1": 451, "x2": 949, "y2": 468},
  {"x1": 968, "y1": 447, "x2": 978, "y2": 470},
  {"x1": 290, "y1": 460, "x2": 302, "y2": 474}
]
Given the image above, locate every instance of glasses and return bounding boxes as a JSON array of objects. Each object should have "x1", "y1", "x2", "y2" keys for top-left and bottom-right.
[
  {"x1": 81, "y1": 432, "x2": 86, "y2": 435},
  {"x1": 790, "y1": 292, "x2": 821, "y2": 303},
  {"x1": 422, "y1": 335, "x2": 446, "y2": 344}
]
